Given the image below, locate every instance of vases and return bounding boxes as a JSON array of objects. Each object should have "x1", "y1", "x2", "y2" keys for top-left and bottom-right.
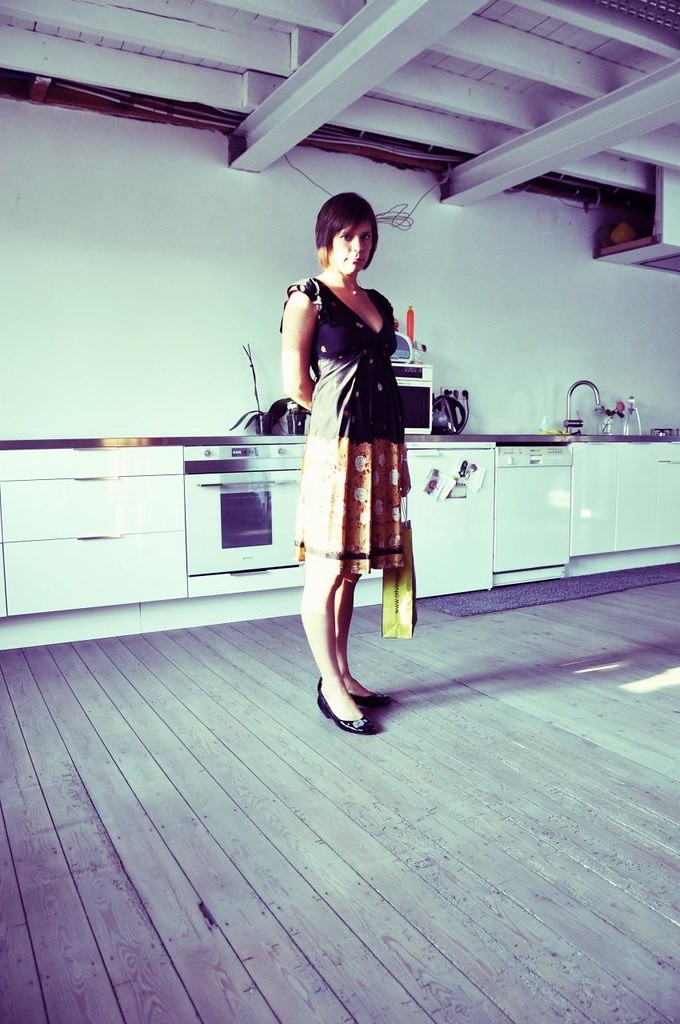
[{"x1": 603, "y1": 419, "x2": 614, "y2": 435}]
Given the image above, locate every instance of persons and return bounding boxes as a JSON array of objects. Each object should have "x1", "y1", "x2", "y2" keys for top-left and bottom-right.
[{"x1": 280, "y1": 191, "x2": 412, "y2": 735}]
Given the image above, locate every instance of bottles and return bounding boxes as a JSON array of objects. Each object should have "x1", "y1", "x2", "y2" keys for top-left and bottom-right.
[{"x1": 623, "y1": 396, "x2": 641, "y2": 435}]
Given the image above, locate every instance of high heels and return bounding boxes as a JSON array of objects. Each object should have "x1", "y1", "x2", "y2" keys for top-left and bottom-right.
[
  {"x1": 318, "y1": 689, "x2": 379, "y2": 735},
  {"x1": 317, "y1": 675, "x2": 392, "y2": 709}
]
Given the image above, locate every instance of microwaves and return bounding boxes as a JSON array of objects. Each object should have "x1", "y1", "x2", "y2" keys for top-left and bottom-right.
[{"x1": 391, "y1": 364, "x2": 433, "y2": 435}]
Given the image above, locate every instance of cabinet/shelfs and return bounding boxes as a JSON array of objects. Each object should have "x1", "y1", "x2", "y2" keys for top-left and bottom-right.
[
  {"x1": 570, "y1": 441, "x2": 679, "y2": 558},
  {"x1": 0, "y1": 446, "x2": 188, "y2": 617}
]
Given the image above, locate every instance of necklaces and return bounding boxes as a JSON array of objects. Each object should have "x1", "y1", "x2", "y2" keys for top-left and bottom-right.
[{"x1": 349, "y1": 289, "x2": 357, "y2": 295}]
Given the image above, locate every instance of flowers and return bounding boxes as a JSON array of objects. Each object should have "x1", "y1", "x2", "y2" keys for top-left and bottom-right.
[{"x1": 594, "y1": 401, "x2": 625, "y2": 420}]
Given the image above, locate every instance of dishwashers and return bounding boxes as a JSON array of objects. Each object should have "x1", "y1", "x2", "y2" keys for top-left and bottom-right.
[{"x1": 493, "y1": 446, "x2": 570, "y2": 574}]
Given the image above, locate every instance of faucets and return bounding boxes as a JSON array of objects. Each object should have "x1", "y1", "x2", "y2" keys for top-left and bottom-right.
[{"x1": 563, "y1": 380, "x2": 601, "y2": 435}]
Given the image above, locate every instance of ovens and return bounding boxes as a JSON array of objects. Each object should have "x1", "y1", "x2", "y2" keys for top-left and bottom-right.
[{"x1": 183, "y1": 444, "x2": 306, "y2": 576}]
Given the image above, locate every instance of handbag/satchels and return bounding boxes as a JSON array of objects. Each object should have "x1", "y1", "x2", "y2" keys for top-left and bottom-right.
[{"x1": 380, "y1": 493, "x2": 418, "y2": 640}]
